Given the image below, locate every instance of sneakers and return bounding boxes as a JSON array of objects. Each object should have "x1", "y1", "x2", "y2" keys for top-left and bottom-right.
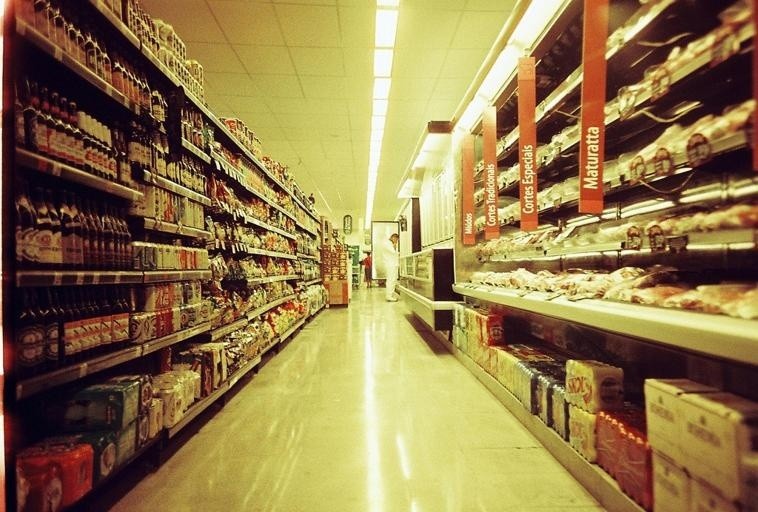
[{"x1": 386, "y1": 297, "x2": 396, "y2": 302}]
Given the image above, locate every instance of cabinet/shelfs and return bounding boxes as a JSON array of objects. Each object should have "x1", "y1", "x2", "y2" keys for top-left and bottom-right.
[
  {"x1": 2, "y1": 0, "x2": 326, "y2": 512},
  {"x1": 399, "y1": 247, "x2": 464, "y2": 331},
  {"x1": 448, "y1": 0, "x2": 755, "y2": 511}
]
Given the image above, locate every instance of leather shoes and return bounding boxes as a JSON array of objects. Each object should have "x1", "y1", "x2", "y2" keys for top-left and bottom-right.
[
  {"x1": 367, "y1": 286, "x2": 370, "y2": 288},
  {"x1": 370, "y1": 286, "x2": 373, "y2": 288}
]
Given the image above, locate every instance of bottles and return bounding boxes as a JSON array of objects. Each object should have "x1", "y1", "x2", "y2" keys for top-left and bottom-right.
[
  {"x1": 132, "y1": 280, "x2": 214, "y2": 345},
  {"x1": 15, "y1": 371, "x2": 201, "y2": 512},
  {"x1": 12, "y1": 70, "x2": 128, "y2": 186},
  {"x1": 133, "y1": 242, "x2": 211, "y2": 270},
  {"x1": 130, "y1": 103, "x2": 213, "y2": 197},
  {"x1": 16, "y1": 167, "x2": 132, "y2": 271},
  {"x1": 14, "y1": 286, "x2": 130, "y2": 380},
  {"x1": 11, "y1": 1, "x2": 173, "y2": 123}
]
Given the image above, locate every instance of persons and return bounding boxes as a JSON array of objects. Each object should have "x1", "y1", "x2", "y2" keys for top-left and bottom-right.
[
  {"x1": 361, "y1": 252, "x2": 372, "y2": 289},
  {"x1": 383, "y1": 234, "x2": 400, "y2": 302}
]
